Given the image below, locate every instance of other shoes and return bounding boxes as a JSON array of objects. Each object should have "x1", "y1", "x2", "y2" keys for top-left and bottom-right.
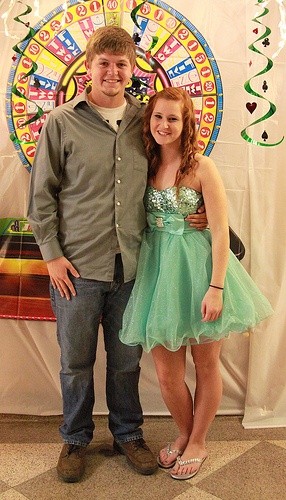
[
  {"x1": 112, "y1": 439, "x2": 158, "y2": 475},
  {"x1": 57, "y1": 443, "x2": 86, "y2": 483}
]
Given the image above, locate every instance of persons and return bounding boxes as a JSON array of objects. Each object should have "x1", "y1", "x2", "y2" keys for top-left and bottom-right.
[
  {"x1": 24, "y1": 26, "x2": 208, "y2": 483},
  {"x1": 118, "y1": 87, "x2": 274, "y2": 481}
]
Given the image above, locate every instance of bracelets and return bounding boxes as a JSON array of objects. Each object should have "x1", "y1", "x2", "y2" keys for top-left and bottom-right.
[{"x1": 209, "y1": 283, "x2": 224, "y2": 291}]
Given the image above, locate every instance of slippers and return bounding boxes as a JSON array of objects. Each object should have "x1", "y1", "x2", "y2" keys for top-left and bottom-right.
[
  {"x1": 156, "y1": 443, "x2": 183, "y2": 468},
  {"x1": 170, "y1": 454, "x2": 208, "y2": 480}
]
